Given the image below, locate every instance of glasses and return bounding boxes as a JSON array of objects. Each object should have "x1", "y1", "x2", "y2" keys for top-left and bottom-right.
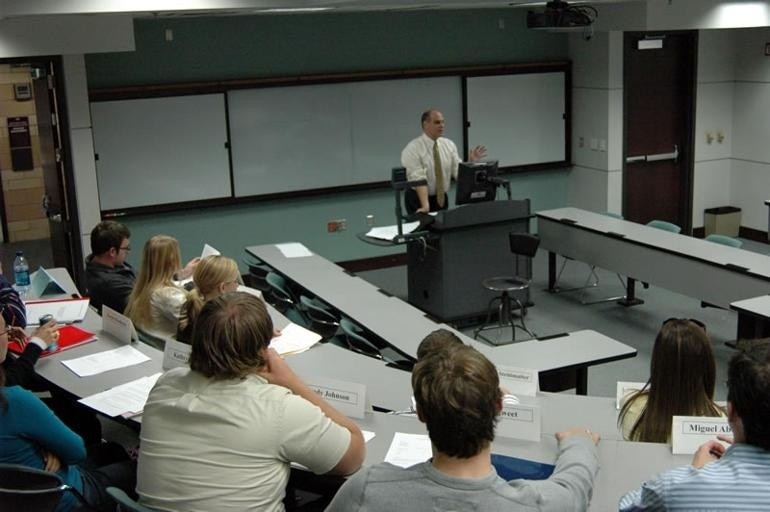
[
  {"x1": 1, "y1": 326, "x2": 14, "y2": 338},
  {"x1": 119, "y1": 243, "x2": 132, "y2": 251},
  {"x1": 663, "y1": 317, "x2": 708, "y2": 336}
]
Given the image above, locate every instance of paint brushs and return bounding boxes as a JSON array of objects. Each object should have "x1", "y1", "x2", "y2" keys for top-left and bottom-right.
[{"x1": 104, "y1": 212, "x2": 125, "y2": 217}]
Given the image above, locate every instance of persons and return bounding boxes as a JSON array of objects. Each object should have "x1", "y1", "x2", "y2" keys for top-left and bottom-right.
[
  {"x1": 401, "y1": 111, "x2": 487, "y2": 223},
  {"x1": 4, "y1": 321, "x2": 66, "y2": 389},
  {"x1": 408, "y1": 327, "x2": 519, "y2": 411},
  {"x1": 0, "y1": 261, "x2": 27, "y2": 330},
  {"x1": 134, "y1": 291, "x2": 366, "y2": 512},
  {"x1": 124, "y1": 233, "x2": 187, "y2": 341},
  {"x1": 0, "y1": 313, "x2": 136, "y2": 512},
  {"x1": 619, "y1": 337, "x2": 770, "y2": 511},
  {"x1": 177, "y1": 255, "x2": 240, "y2": 345},
  {"x1": 84, "y1": 221, "x2": 138, "y2": 316},
  {"x1": 616, "y1": 317, "x2": 728, "y2": 443},
  {"x1": 324, "y1": 342, "x2": 600, "y2": 511}
]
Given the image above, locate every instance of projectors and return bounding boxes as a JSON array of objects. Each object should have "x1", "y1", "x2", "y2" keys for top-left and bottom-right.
[{"x1": 526, "y1": 2, "x2": 597, "y2": 32}]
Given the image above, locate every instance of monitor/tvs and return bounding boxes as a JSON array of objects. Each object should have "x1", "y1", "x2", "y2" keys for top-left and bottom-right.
[{"x1": 455, "y1": 161, "x2": 498, "y2": 205}]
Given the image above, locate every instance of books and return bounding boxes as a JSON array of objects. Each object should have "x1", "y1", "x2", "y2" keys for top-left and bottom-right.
[
  {"x1": 24, "y1": 298, "x2": 90, "y2": 325},
  {"x1": 8, "y1": 325, "x2": 97, "y2": 359}
]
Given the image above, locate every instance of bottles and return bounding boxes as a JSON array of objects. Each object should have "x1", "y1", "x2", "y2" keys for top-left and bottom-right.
[
  {"x1": 14, "y1": 251, "x2": 30, "y2": 300},
  {"x1": 40, "y1": 315, "x2": 58, "y2": 353}
]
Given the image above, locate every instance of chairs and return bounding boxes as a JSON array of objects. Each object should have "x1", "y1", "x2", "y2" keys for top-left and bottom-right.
[
  {"x1": 295, "y1": 295, "x2": 338, "y2": 342},
  {"x1": 242, "y1": 260, "x2": 272, "y2": 294},
  {"x1": 0, "y1": 463, "x2": 86, "y2": 510},
  {"x1": 697, "y1": 234, "x2": 744, "y2": 311},
  {"x1": 629, "y1": 220, "x2": 681, "y2": 291},
  {"x1": 332, "y1": 319, "x2": 388, "y2": 362},
  {"x1": 264, "y1": 272, "x2": 309, "y2": 323},
  {"x1": 552, "y1": 212, "x2": 627, "y2": 307},
  {"x1": 473, "y1": 233, "x2": 541, "y2": 345}
]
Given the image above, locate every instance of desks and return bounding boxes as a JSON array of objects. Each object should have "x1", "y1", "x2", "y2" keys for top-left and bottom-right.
[
  {"x1": 177, "y1": 278, "x2": 727, "y2": 441},
  {"x1": 8, "y1": 279, "x2": 721, "y2": 511},
  {"x1": 537, "y1": 207, "x2": 770, "y2": 363},
  {"x1": 395, "y1": 198, "x2": 542, "y2": 329},
  {"x1": 245, "y1": 242, "x2": 638, "y2": 394}
]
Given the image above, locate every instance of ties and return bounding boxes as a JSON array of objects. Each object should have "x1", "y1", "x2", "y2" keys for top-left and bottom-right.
[{"x1": 433, "y1": 140, "x2": 445, "y2": 207}]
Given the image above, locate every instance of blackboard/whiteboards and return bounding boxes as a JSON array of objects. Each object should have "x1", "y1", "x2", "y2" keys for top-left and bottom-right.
[{"x1": 89, "y1": 61, "x2": 571, "y2": 219}]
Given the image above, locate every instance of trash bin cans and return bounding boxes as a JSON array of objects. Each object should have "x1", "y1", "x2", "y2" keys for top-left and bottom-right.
[{"x1": 705, "y1": 205, "x2": 744, "y2": 238}]
